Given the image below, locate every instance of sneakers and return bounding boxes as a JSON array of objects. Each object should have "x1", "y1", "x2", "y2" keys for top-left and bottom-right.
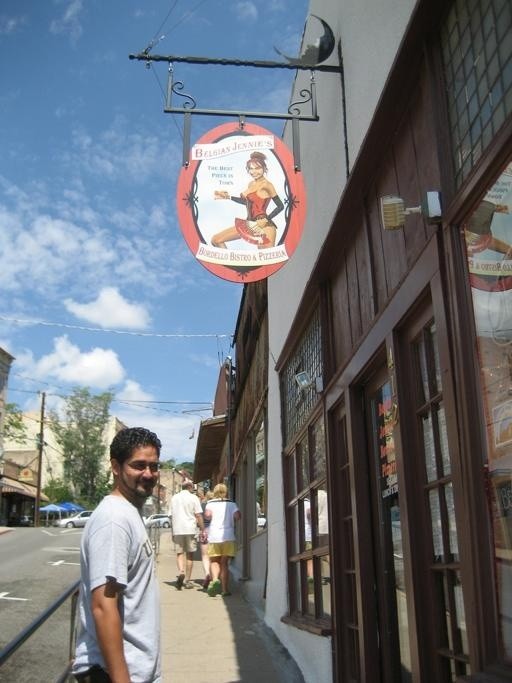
[
  {"x1": 203, "y1": 574, "x2": 231, "y2": 596},
  {"x1": 176, "y1": 572, "x2": 193, "y2": 590}
]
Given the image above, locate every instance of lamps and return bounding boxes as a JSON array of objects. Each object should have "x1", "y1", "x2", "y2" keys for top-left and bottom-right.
[
  {"x1": 293, "y1": 371, "x2": 323, "y2": 394},
  {"x1": 380, "y1": 192, "x2": 440, "y2": 232}
]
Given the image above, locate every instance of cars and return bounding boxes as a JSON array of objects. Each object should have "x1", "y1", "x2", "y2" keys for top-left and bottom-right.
[
  {"x1": 143, "y1": 514, "x2": 172, "y2": 528},
  {"x1": 56, "y1": 510, "x2": 94, "y2": 528}
]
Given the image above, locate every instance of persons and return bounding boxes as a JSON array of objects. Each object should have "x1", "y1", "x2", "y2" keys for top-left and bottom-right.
[
  {"x1": 71, "y1": 427, "x2": 162, "y2": 682},
  {"x1": 210, "y1": 151, "x2": 284, "y2": 249},
  {"x1": 303, "y1": 489, "x2": 330, "y2": 579},
  {"x1": 466, "y1": 206, "x2": 512, "y2": 260},
  {"x1": 168, "y1": 480, "x2": 241, "y2": 597}
]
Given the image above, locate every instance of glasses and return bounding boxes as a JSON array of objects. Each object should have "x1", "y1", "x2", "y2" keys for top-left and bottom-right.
[{"x1": 123, "y1": 458, "x2": 161, "y2": 472}]
